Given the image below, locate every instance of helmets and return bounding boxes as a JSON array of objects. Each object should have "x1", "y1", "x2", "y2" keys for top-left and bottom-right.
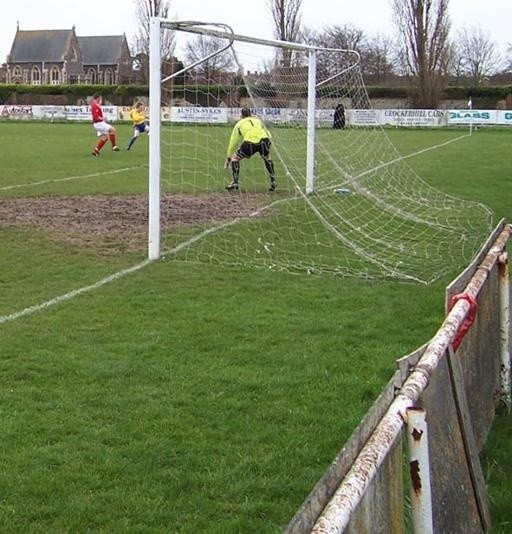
[{"x1": 241, "y1": 108, "x2": 251, "y2": 117}]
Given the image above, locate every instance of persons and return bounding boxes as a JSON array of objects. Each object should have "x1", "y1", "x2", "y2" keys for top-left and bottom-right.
[
  {"x1": 125, "y1": 102, "x2": 150, "y2": 150},
  {"x1": 224, "y1": 108, "x2": 277, "y2": 191},
  {"x1": 92, "y1": 93, "x2": 120, "y2": 157}
]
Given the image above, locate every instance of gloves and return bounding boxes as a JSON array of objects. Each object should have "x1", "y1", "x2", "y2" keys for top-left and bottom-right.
[{"x1": 224, "y1": 157, "x2": 231, "y2": 169}]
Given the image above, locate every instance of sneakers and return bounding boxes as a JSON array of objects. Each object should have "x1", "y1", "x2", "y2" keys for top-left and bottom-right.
[
  {"x1": 224, "y1": 182, "x2": 240, "y2": 191},
  {"x1": 268, "y1": 181, "x2": 278, "y2": 191}
]
